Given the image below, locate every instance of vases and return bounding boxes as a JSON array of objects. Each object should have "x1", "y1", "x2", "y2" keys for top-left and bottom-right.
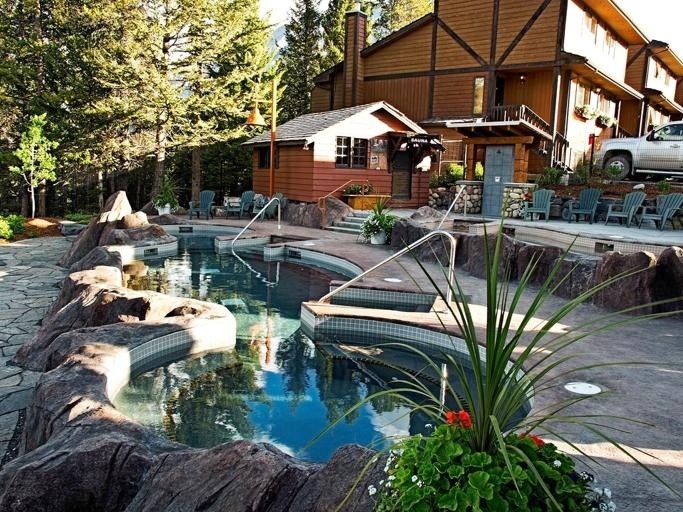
[{"x1": 370, "y1": 228, "x2": 386, "y2": 244}]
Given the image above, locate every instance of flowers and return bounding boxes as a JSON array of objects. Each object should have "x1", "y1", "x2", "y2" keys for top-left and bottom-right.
[
  {"x1": 357, "y1": 188, "x2": 396, "y2": 242},
  {"x1": 297, "y1": 197, "x2": 683, "y2": 512}
]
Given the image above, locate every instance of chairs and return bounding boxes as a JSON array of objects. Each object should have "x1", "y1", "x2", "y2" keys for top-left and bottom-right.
[
  {"x1": 187, "y1": 190, "x2": 215, "y2": 220},
  {"x1": 225, "y1": 190, "x2": 283, "y2": 220},
  {"x1": 523, "y1": 188, "x2": 682, "y2": 232}
]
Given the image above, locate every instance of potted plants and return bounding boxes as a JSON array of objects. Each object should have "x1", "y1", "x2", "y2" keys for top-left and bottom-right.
[
  {"x1": 342, "y1": 180, "x2": 390, "y2": 210},
  {"x1": 575, "y1": 104, "x2": 618, "y2": 128},
  {"x1": 148, "y1": 172, "x2": 181, "y2": 216}
]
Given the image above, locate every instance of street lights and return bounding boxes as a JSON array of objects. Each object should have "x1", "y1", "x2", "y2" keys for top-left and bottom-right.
[{"x1": 245, "y1": 78, "x2": 277, "y2": 201}]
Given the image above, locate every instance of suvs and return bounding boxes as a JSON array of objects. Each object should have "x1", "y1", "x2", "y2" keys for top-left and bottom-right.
[{"x1": 593, "y1": 121, "x2": 683, "y2": 181}]
